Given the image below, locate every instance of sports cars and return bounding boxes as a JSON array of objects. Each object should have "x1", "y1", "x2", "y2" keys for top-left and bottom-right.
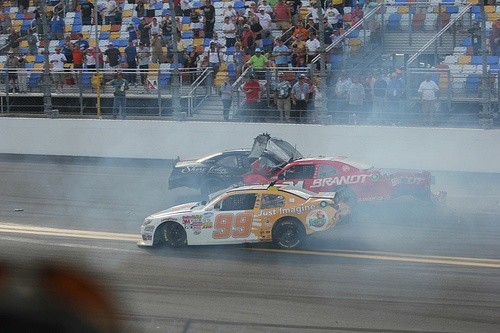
[
  {"x1": 168, "y1": 135, "x2": 432, "y2": 207},
  {"x1": 136, "y1": 180, "x2": 351, "y2": 249}
]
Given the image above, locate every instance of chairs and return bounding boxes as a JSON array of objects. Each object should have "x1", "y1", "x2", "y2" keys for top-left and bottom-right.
[{"x1": 0, "y1": 0, "x2": 500, "y2": 93}]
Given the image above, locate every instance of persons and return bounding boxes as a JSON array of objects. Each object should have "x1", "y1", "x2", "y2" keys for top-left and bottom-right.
[
  {"x1": 467, "y1": 18, "x2": 500, "y2": 59},
  {"x1": 310, "y1": 79, "x2": 328, "y2": 123},
  {"x1": 244, "y1": 75, "x2": 261, "y2": 121},
  {"x1": 106, "y1": 72, "x2": 129, "y2": 119},
  {"x1": 386, "y1": 76, "x2": 406, "y2": 124},
  {"x1": 290, "y1": 77, "x2": 310, "y2": 122},
  {"x1": 0, "y1": 0, "x2": 363, "y2": 99},
  {"x1": 417, "y1": 74, "x2": 440, "y2": 127},
  {"x1": 219, "y1": 79, "x2": 233, "y2": 119},
  {"x1": 370, "y1": 75, "x2": 389, "y2": 122},
  {"x1": 347, "y1": 77, "x2": 365, "y2": 123},
  {"x1": 273, "y1": 74, "x2": 293, "y2": 123}
]
